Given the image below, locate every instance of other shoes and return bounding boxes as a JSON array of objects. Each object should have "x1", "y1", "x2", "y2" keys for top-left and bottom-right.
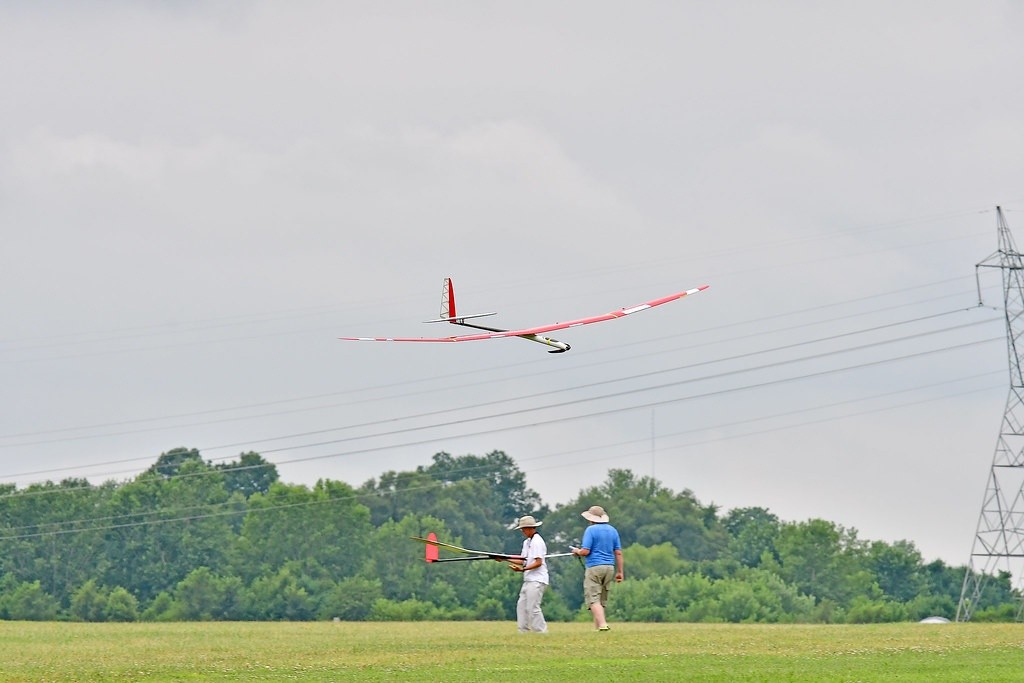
[{"x1": 600, "y1": 627, "x2": 610, "y2": 631}]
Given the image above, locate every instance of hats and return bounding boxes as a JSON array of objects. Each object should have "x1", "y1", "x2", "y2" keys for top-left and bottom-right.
[
  {"x1": 514, "y1": 516, "x2": 543, "y2": 530},
  {"x1": 581, "y1": 506, "x2": 610, "y2": 523}
]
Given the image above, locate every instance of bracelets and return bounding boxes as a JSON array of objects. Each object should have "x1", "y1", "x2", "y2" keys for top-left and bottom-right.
[{"x1": 523, "y1": 565, "x2": 526, "y2": 572}]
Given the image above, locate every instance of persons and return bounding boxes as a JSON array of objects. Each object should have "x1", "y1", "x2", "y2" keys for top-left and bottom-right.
[
  {"x1": 572, "y1": 506, "x2": 623, "y2": 631},
  {"x1": 495, "y1": 516, "x2": 549, "y2": 633}
]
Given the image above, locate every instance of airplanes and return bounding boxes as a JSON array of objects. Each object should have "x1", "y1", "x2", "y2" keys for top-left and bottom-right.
[
  {"x1": 410, "y1": 533, "x2": 579, "y2": 562},
  {"x1": 337, "y1": 278, "x2": 708, "y2": 355}
]
